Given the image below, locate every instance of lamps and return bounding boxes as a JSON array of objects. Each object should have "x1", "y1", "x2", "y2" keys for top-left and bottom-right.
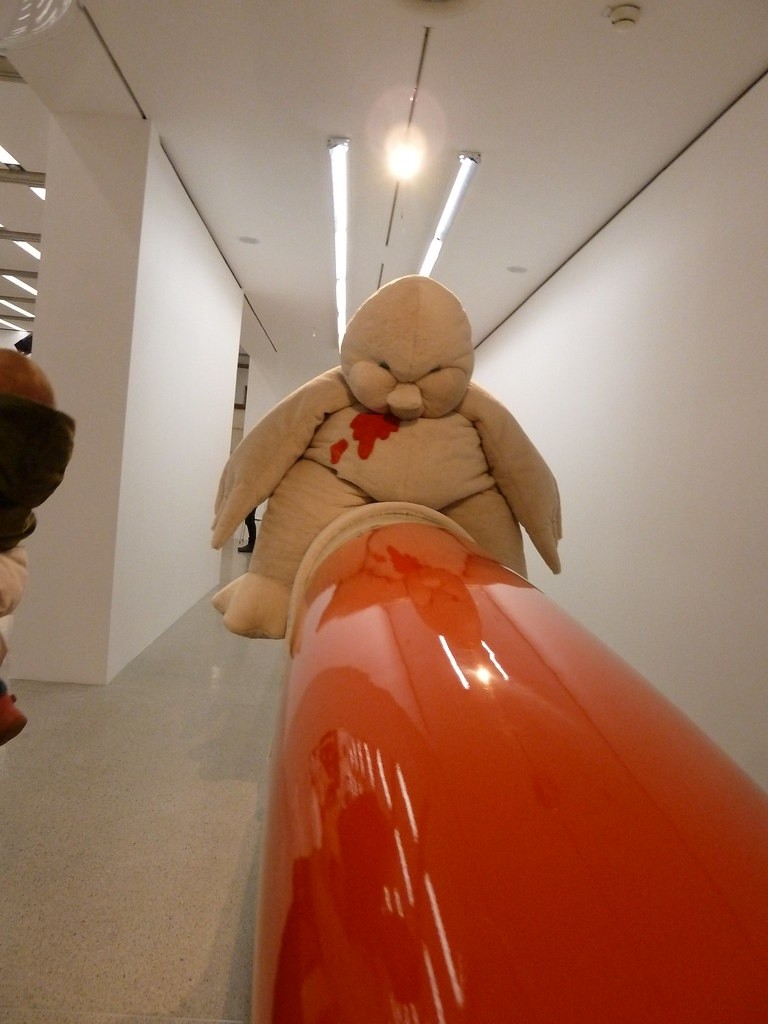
[
  {"x1": 417, "y1": 150, "x2": 480, "y2": 276},
  {"x1": 327, "y1": 137, "x2": 348, "y2": 354}
]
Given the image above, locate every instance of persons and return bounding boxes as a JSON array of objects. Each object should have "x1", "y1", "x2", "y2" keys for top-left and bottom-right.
[{"x1": 238, "y1": 509, "x2": 257, "y2": 553}]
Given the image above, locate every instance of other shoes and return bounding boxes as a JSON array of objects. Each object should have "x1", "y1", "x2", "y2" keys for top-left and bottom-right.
[{"x1": 0, "y1": 691, "x2": 28, "y2": 747}]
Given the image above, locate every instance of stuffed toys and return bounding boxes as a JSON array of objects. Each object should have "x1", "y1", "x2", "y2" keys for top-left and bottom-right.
[
  {"x1": 210, "y1": 275, "x2": 564, "y2": 639},
  {"x1": 0, "y1": 348, "x2": 77, "y2": 745}
]
